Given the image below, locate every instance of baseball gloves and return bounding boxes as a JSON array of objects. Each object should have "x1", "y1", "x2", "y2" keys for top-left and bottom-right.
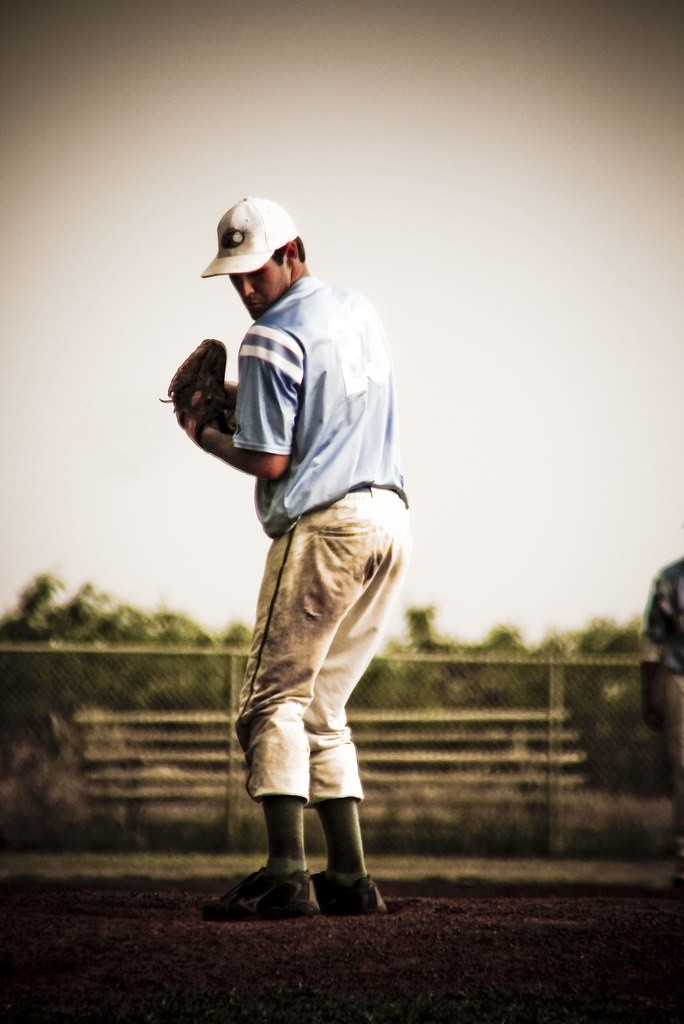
[{"x1": 156, "y1": 337, "x2": 238, "y2": 453}]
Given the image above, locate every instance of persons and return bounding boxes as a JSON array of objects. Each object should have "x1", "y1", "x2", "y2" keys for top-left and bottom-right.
[
  {"x1": 168, "y1": 195, "x2": 411, "y2": 919},
  {"x1": 637, "y1": 558, "x2": 684, "y2": 880}
]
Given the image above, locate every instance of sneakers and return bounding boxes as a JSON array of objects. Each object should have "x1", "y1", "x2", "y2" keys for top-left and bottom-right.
[
  {"x1": 201, "y1": 867, "x2": 321, "y2": 921},
  {"x1": 308, "y1": 871, "x2": 387, "y2": 916}
]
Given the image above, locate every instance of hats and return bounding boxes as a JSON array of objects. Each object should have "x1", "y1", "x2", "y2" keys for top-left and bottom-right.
[{"x1": 201, "y1": 198, "x2": 298, "y2": 278}]
